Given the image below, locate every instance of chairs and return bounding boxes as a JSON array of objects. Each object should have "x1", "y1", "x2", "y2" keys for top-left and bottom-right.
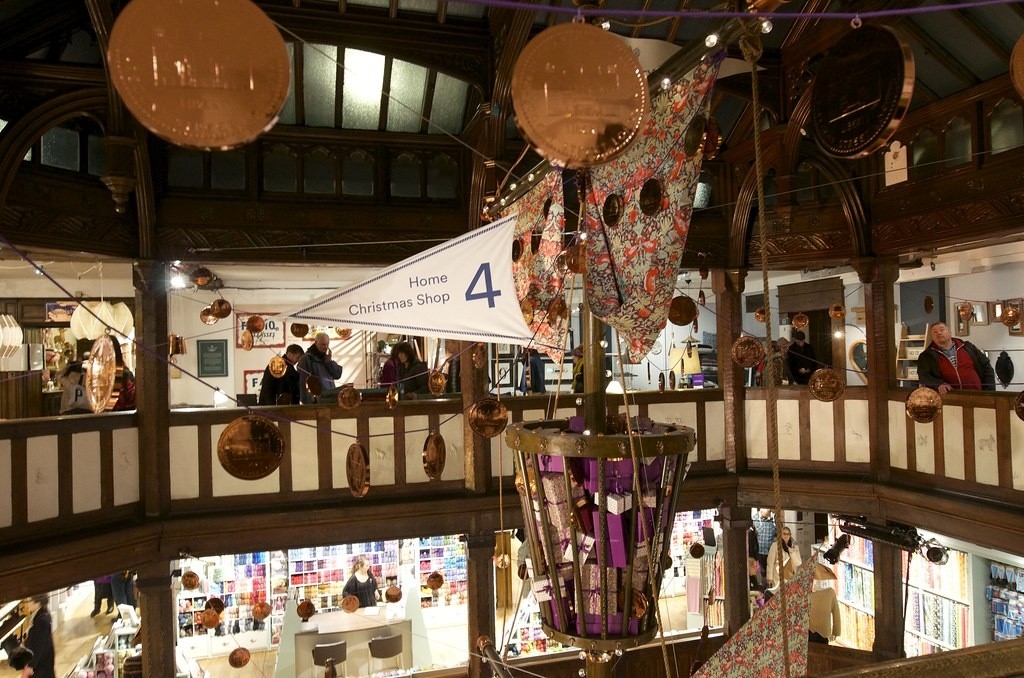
[
  {"x1": 368, "y1": 633, "x2": 404, "y2": 678},
  {"x1": 312, "y1": 641, "x2": 347, "y2": 678}
]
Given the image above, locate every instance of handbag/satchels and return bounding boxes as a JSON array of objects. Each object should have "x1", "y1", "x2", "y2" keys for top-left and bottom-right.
[
  {"x1": 114, "y1": 367, "x2": 137, "y2": 411},
  {"x1": 68, "y1": 374, "x2": 93, "y2": 412}
]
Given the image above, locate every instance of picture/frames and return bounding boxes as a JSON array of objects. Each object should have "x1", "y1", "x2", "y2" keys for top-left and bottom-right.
[{"x1": 492, "y1": 313, "x2": 576, "y2": 388}]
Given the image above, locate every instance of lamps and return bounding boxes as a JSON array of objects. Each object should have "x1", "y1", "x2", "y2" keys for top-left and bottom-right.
[
  {"x1": 682, "y1": 332, "x2": 700, "y2": 343},
  {"x1": 70, "y1": 258, "x2": 133, "y2": 340},
  {"x1": 605, "y1": 356, "x2": 624, "y2": 394}
]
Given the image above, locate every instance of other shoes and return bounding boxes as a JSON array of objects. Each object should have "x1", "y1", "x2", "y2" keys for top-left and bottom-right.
[
  {"x1": 111, "y1": 617, "x2": 118, "y2": 622},
  {"x1": 105, "y1": 605, "x2": 115, "y2": 616},
  {"x1": 89, "y1": 609, "x2": 98, "y2": 618},
  {"x1": 91, "y1": 610, "x2": 100, "y2": 617},
  {"x1": 106, "y1": 606, "x2": 114, "y2": 613}
]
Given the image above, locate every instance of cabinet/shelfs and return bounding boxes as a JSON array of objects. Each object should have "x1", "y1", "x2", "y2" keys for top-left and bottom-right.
[
  {"x1": 896, "y1": 323, "x2": 931, "y2": 388},
  {"x1": 288, "y1": 539, "x2": 398, "y2": 613},
  {"x1": 173, "y1": 552, "x2": 271, "y2": 658},
  {"x1": 63, "y1": 604, "x2": 142, "y2": 678},
  {"x1": 46, "y1": 580, "x2": 94, "y2": 634},
  {"x1": 810, "y1": 514, "x2": 992, "y2": 658},
  {"x1": 418, "y1": 534, "x2": 469, "y2": 609}
]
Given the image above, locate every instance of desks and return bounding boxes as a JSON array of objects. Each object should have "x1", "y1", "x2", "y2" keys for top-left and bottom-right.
[{"x1": 272, "y1": 588, "x2": 432, "y2": 678}]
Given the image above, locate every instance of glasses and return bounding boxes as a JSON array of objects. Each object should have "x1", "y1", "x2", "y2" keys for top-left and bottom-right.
[{"x1": 783, "y1": 532, "x2": 790, "y2": 536}]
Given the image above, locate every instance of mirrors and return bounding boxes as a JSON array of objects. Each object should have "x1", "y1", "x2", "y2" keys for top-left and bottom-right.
[
  {"x1": 849, "y1": 340, "x2": 868, "y2": 386},
  {"x1": 990, "y1": 300, "x2": 1005, "y2": 322},
  {"x1": 970, "y1": 301, "x2": 989, "y2": 326},
  {"x1": 954, "y1": 302, "x2": 968, "y2": 336},
  {"x1": 1007, "y1": 298, "x2": 1024, "y2": 335}
]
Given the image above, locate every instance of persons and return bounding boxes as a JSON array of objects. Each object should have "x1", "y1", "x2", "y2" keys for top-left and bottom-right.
[
  {"x1": 112, "y1": 569, "x2": 137, "y2": 622},
  {"x1": 56, "y1": 363, "x2": 95, "y2": 414},
  {"x1": 258, "y1": 344, "x2": 304, "y2": 405},
  {"x1": 91, "y1": 576, "x2": 115, "y2": 617},
  {"x1": 381, "y1": 345, "x2": 397, "y2": 389},
  {"x1": 572, "y1": 343, "x2": 584, "y2": 393},
  {"x1": 761, "y1": 341, "x2": 782, "y2": 387},
  {"x1": 113, "y1": 361, "x2": 136, "y2": 412},
  {"x1": 777, "y1": 337, "x2": 798, "y2": 386},
  {"x1": 766, "y1": 526, "x2": 802, "y2": 593},
  {"x1": 444, "y1": 340, "x2": 461, "y2": 392},
  {"x1": 342, "y1": 557, "x2": 380, "y2": 608},
  {"x1": 788, "y1": 332, "x2": 817, "y2": 385},
  {"x1": 807, "y1": 587, "x2": 840, "y2": 645},
  {"x1": 396, "y1": 342, "x2": 431, "y2": 400},
  {"x1": 23, "y1": 594, "x2": 56, "y2": 678},
  {"x1": 752, "y1": 507, "x2": 776, "y2": 572},
  {"x1": 748, "y1": 526, "x2": 759, "y2": 591},
  {"x1": 521, "y1": 348, "x2": 545, "y2": 395},
  {"x1": 298, "y1": 334, "x2": 342, "y2": 406},
  {"x1": 918, "y1": 322, "x2": 996, "y2": 394}
]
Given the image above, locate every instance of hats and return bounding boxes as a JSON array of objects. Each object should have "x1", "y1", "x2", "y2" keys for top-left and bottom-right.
[
  {"x1": 571, "y1": 343, "x2": 583, "y2": 356},
  {"x1": 777, "y1": 337, "x2": 791, "y2": 347}
]
